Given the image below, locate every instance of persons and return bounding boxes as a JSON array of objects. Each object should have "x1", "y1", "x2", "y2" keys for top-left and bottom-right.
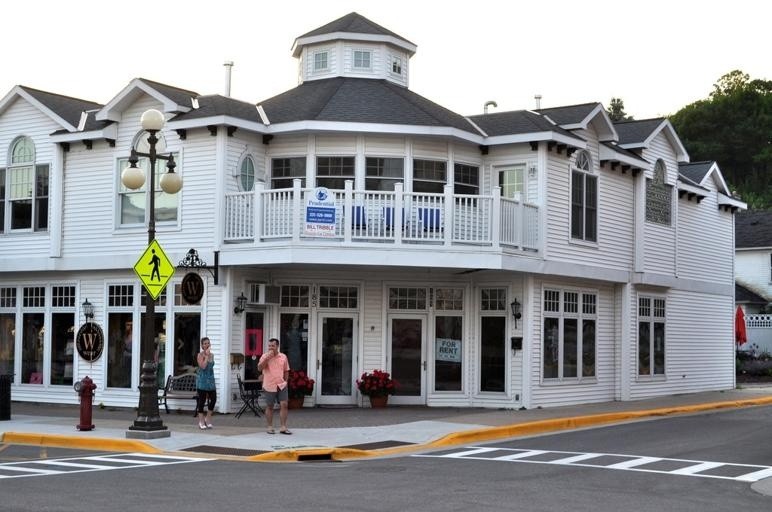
[
  {"x1": 153, "y1": 319, "x2": 167, "y2": 390},
  {"x1": 195, "y1": 337, "x2": 217, "y2": 429},
  {"x1": 258, "y1": 336, "x2": 292, "y2": 434}
]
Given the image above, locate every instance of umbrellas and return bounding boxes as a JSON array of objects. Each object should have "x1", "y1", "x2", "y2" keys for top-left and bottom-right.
[{"x1": 735, "y1": 304, "x2": 747, "y2": 351}]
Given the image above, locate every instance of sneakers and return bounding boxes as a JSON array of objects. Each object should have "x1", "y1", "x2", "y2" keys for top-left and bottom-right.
[
  {"x1": 205, "y1": 418, "x2": 213, "y2": 428},
  {"x1": 278, "y1": 428, "x2": 291, "y2": 435},
  {"x1": 266, "y1": 425, "x2": 275, "y2": 435},
  {"x1": 199, "y1": 419, "x2": 206, "y2": 430}
]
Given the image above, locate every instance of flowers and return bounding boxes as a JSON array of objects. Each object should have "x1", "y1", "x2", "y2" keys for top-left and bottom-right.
[
  {"x1": 356, "y1": 369, "x2": 402, "y2": 396},
  {"x1": 287, "y1": 368, "x2": 315, "y2": 400}
]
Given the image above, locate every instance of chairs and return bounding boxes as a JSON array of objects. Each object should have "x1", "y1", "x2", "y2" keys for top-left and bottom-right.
[
  {"x1": 416, "y1": 207, "x2": 444, "y2": 244},
  {"x1": 342, "y1": 205, "x2": 372, "y2": 242},
  {"x1": 381, "y1": 206, "x2": 410, "y2": 244},
  {"x1": 235, "y1": 373, "x2": 266, "y2": 420}
]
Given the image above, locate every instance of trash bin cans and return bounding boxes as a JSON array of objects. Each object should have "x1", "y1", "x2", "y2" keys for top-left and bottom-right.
[{"x1": 0, "y1": 374, "x2": 17, "y2": 420}]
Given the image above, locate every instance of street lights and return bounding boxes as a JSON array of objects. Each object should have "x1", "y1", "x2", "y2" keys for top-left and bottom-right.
[{"x1": 121, "y1": 110, "x2": 182, "y2": 431}]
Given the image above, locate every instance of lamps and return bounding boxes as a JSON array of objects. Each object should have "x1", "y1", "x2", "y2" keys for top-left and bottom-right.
[
  {"x1": 511, "y1": 297, "x2": 522, "y2": 329},
  {"x1": 82, "y1": 298, "x2": 94, "y2": 324},
  {"x1": 234, "y1": 292, "x2": 248, "y2": 313}
]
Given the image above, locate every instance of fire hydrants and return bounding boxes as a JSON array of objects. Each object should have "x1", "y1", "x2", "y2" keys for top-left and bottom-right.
[{"x1": 74, "y1": 376, "x2": 97, "y2": 431}]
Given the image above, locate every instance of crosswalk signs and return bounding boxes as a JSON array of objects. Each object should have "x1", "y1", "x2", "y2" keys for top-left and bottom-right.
[{"x1": 133, "y1": 239, "x2": 175, "y2": 301}]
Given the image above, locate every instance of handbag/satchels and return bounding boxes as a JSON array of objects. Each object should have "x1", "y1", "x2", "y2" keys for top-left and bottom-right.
[{"x1": 28, "y1": 372, "x2": 44, "y2": 385}]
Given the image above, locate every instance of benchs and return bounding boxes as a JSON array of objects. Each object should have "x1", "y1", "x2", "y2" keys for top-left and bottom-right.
[{"x1": 156, "y1": 372, "x2": 210, "y2": 417}]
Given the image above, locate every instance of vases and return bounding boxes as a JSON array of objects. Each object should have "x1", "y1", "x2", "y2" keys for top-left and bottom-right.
[
  {"x1": 286, "y1": 394, "x2": 303, "y2": 409},
  {"x1": 369, "y1": 393, "x2": 388, "y2": 407}
]
